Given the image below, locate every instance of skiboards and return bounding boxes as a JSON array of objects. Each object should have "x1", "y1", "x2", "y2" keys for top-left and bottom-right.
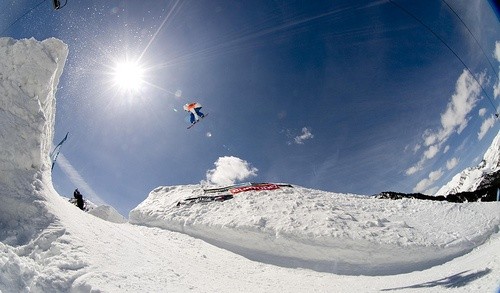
[{"x1": 177, "y1": 182, "x2": 294, "y2": 207}]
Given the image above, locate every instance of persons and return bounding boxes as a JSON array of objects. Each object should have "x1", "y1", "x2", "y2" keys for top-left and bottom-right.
[{"x1": 183, "y1": 101, "x2": 204, "y2": 124}]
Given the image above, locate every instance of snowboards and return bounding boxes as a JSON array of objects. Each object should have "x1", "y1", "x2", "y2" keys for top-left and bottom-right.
[{"x1": 186, "y1": 114, "x2": 209, "y2": 130}]
